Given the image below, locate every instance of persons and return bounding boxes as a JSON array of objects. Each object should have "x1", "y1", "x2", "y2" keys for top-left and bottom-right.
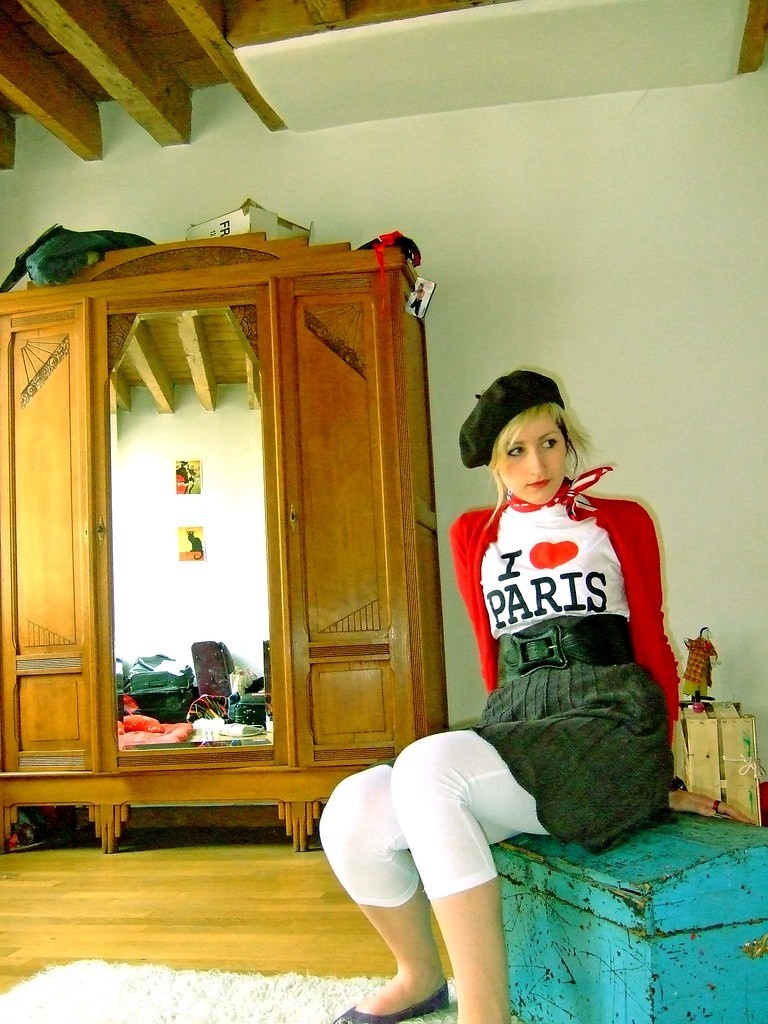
[
  {"x1": 320, "y1": 369, "x2": 680, "y2": 1023},
  {"x1": 410, "y1": 283, "x2": 425, "y2": 316},
  {"x1": 682, "y1": 628, "x2": 718, "y2": 700}
]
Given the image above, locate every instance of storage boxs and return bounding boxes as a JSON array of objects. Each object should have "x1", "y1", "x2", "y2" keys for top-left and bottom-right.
[
  {"x1": 186, "y1": 197, "x2": 317, "y2": 248},
  {"x1": 488, "y1": 810, "x2": 768, "y2": 1024}
]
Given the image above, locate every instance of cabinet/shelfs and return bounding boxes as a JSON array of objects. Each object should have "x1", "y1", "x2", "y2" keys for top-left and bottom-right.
[{"x1": 0, "y1": 232, "x2": 450, "y2": 854}]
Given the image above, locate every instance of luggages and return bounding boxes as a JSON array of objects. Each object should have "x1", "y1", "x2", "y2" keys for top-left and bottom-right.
[{"x1": 228, "y1": 694, "x2": 266, "y2": 727}]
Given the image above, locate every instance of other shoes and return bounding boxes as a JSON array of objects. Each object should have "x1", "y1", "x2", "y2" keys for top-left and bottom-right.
[{"x1": 334, "y1": 977, "x2": 450, "y2": 1024}]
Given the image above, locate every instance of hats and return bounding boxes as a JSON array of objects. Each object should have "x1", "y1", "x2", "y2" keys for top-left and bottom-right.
[{"x1": 459, "y1": 370, "x2": 566, "y2": 468}]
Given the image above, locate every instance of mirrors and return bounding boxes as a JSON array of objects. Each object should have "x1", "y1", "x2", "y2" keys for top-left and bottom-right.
[{"x1": 94, "y1": 287, "x2": 277, "y2": 757}]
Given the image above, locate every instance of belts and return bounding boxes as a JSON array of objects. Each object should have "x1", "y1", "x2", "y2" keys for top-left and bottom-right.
[{"x1": 495, "y1": 614, "x2": 636, "y2": 690}]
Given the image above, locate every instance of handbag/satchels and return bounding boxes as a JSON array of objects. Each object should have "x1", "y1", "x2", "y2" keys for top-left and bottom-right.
[{"x1": 131, "y1": 667, "x2": 194, "y2": 691}]
